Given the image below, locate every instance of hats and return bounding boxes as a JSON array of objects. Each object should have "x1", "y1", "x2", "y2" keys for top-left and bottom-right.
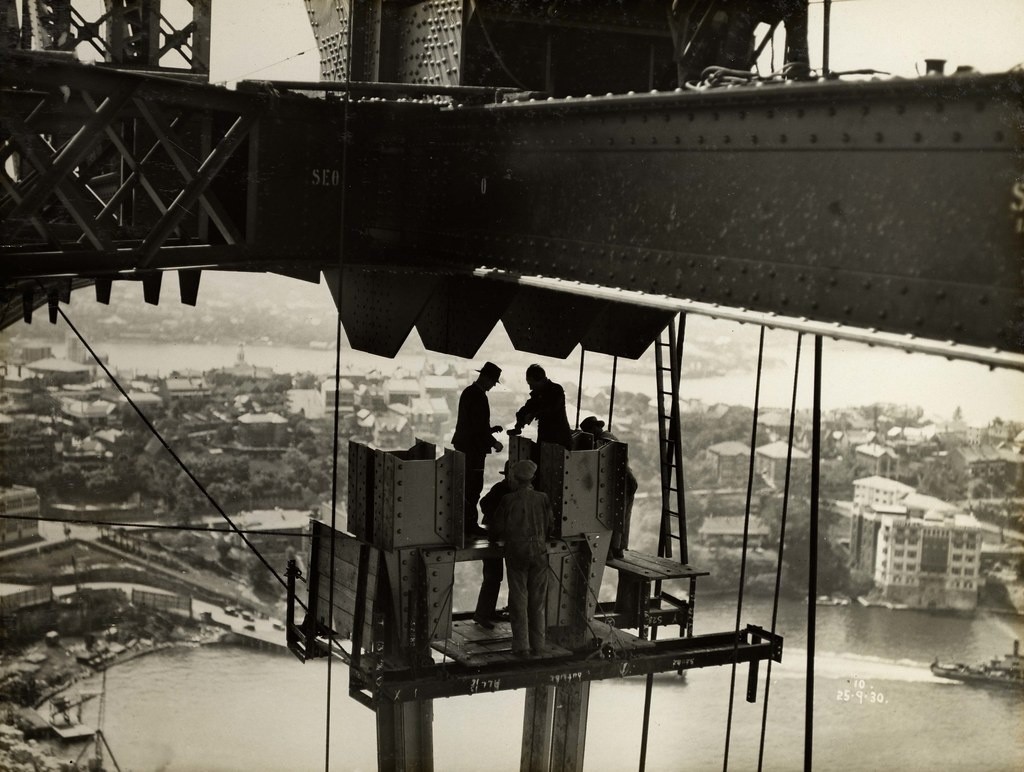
[
  {"x1": 580, "y1": 417, "x2": 605, "y2": 433},
  {"x1": 510, "y1": 459, "x2": 538, "y2": 479},
  {"x1": 498, "y1": 461, "x2": 508, "y2": 474},
  {"x1": 474, "y1": 363, "x2": 501, "y2": 383}
]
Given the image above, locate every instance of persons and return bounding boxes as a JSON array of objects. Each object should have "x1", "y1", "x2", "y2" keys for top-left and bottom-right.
[
  {"x1": 579, "y1": 416, "x2": 639, "y2": 561},
  {"x1": 488, "y1": 460, "x2": 555, "y2": 658},
  {"x1": 450, "y1": 361, "x2": 504, "y2": 538},
  {"x1": 514, "y1": 363, "x2": 575, "y2": 452},
  {"x1": 472, "y1": 460, "x2": 509, "y2": 630}
]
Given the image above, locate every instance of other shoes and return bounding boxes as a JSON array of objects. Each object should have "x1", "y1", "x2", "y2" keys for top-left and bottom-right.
[
  {"x1": 607, "y1": 550, "x2": 622, "y2": 559},
  {"x1": 488, "y1": 612, "x2": 505, "y2": 620},
  {"x1": 473, "y1": 614, "x2": 496, "y2": 630},
  {"x1": 533, "y1": 650, "x2": 544, "y2": 655},
  {"x1": 509, "y1": 650, "x2": 530, "y2": 657},
  {"x1": 467, "y1": 524, "x2": 484, "y2": 534}
]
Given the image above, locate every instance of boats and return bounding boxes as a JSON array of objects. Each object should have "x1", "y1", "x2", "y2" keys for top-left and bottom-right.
[{"x1": 930, "y1": 639, "x2": 1024, "y2": 689}]
